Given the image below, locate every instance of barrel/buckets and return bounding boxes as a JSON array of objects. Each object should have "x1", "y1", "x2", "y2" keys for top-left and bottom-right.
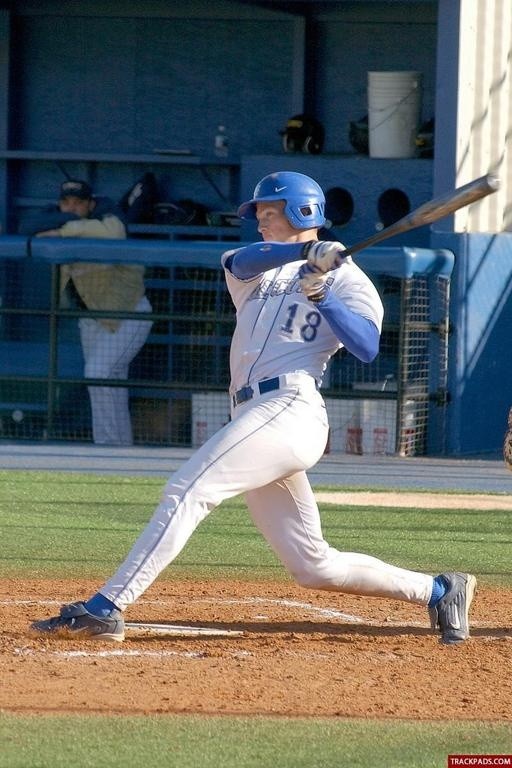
[
  {"x1": 194, "y1": 372, "x2": 418, "y2": 459},
  {"x1": 367, "y1": 71, "x2": 422, "y2": 158}
]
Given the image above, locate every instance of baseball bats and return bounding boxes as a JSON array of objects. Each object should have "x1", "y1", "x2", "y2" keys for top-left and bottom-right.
[{"x1": 300, "y1": 173, "x2": 500, "y2": 278}]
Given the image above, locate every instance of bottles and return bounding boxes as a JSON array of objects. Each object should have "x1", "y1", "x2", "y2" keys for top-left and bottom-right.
[{"x1": 214, "y1": 126, "x2": 230, "y2": 158}]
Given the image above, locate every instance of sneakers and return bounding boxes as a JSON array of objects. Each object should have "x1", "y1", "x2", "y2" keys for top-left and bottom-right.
[
  {"x1": 429, "y1": 571, "x2": 477, "y2": 645},
  {"x1": 29, "y1": 602, "x2": 124, "y2": 643}
]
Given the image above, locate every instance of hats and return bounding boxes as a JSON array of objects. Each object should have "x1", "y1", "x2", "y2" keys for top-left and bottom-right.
[{"x1": 58, "y1": 181, "x2": 92, "y2": 201}]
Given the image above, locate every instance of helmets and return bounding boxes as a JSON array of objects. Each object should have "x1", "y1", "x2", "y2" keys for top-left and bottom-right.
[
  {"x1": 280, "y1": 114, "x2": 324, "y2": 152},
  {"x1": 237, "y1": 173, "x2": 327, "y2": 229}
]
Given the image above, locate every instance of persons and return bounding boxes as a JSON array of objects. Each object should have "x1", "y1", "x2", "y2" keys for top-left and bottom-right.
[
  {"x1": 29, "y1": 171, "x2": 477, "y2": 644},
  {"x1": 19, "y1": 179, "x2": 155, "y2": 445}
]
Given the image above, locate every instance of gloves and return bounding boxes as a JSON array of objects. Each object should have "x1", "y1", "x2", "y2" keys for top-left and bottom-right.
[
  {"x1": 302, "y1": 240, "x2": 348, "y2": 273},
  {"x1": 301, "y1": 273, "x2": 328, "y2": 300}
]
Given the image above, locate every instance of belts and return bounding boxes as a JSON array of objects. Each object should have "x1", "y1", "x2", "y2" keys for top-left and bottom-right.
[{"x1": 232, "y1": 376, "x2": 279, "y2": 406}]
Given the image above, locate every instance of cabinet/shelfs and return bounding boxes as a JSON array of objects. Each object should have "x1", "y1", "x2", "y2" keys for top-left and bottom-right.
[{"x1": 0, "y1": 0, "x2": 461, "y2": 414}]
